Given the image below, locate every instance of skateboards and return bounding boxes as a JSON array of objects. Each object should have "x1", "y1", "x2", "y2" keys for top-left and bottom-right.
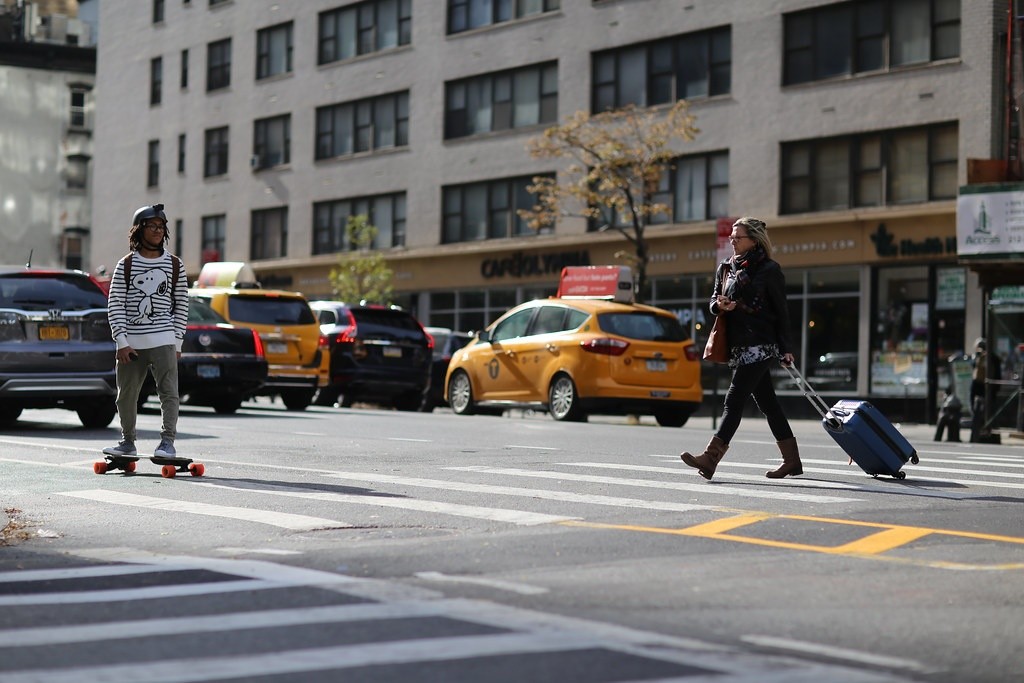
[{"x1": 94, "y1": 452, "x2": 204, "y2": 478}]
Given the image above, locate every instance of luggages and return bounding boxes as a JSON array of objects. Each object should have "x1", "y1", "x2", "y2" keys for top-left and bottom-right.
[{"x1": 781, "y1": 357, "x2": 919, "y2": 481}]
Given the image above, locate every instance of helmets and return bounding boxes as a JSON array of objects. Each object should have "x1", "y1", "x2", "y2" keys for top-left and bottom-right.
[{"x1": 132, "y1": 206, "x2": 168, "y2": 225}]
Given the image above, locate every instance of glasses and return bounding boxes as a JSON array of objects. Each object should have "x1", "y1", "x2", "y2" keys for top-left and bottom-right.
[
  {"x1": 728, "y1": 234, "x2": 749, "y2": 242},
  {"x1": 143, "y1": 224, "x2": 166, "y2": 233}
]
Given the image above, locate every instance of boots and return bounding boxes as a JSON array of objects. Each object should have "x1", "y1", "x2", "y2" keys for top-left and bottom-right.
[
  {"x1": 680, "y1": 434, "x2": 730, "y2": 480},
  {"x1": 766, "y1": 437, "x2": 805, "y2": 479}
]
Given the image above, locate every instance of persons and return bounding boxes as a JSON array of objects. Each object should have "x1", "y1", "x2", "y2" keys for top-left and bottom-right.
[
  {"x1": 969, "y1": 336, "x2": 1002, "y2": 418},
  {"x1": 103, "y1": 205, "x2": 189, "y2": 457},
  {"x1": 681, "y1": 215, "x2": 804, "y2": 481}
]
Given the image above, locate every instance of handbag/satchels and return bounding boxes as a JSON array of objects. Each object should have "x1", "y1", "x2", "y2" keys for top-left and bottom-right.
[{"x1": 703, "y1": 316, "x2": 730, "y2": 365}]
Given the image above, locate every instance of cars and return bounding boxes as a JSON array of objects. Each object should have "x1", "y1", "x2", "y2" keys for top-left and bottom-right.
[
  {"x1": 0, "y1": 248, "x2": 118, "y2": 429},
  {"x1": 308, "y1": 299, "x2": 434, "y2": 410},
  {"x1": 188, "y1": 283, "x2": 330, "y2": 411},
  {"x1": 135, "y1": 298, "x2": 269, "y2": 416},
  {"x1": 419, "y1": 325, "x2": 473, "y2": 398},
  {"x1": 444, "y1": 296, "x2": 703, "y2": 429}
]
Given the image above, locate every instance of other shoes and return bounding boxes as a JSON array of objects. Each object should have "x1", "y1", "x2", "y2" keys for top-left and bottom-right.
[
  {"x1": 154, "y1": 444, "x2": 176, "y2": 457},
  {"x1": 103, "y1": 442, "x2": 137, "y2": 455}
]
